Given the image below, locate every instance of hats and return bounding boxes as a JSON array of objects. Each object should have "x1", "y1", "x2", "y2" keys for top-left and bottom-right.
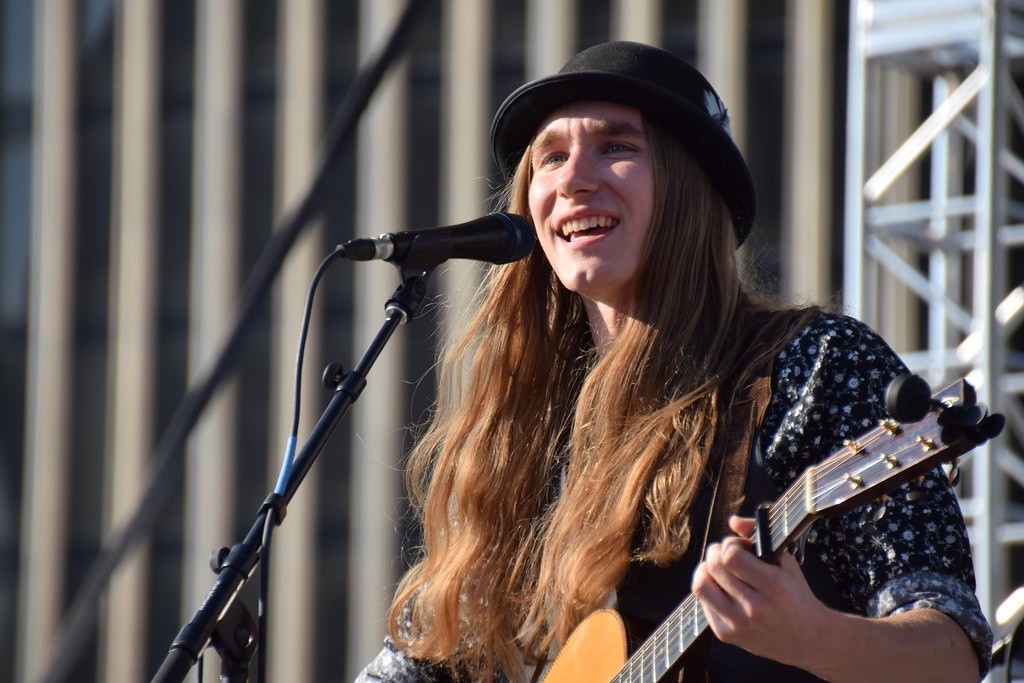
[{"x1": 489, "y1": 40, "x2": 757, "y2": 254}]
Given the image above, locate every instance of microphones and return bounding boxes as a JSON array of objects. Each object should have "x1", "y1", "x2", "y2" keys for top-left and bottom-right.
[{"x1": 336, "y1": 212, "x2": 535, "y2": 266}]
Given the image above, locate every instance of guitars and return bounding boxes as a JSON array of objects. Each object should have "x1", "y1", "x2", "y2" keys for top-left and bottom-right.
[{"x1": 539, "y1": 372, "x2": 1004, "y2": 683}]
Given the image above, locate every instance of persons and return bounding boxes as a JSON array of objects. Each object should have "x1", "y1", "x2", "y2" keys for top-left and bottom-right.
[{"x1": 356, "y1": 41, "x2": 995, "y2": 683}]
